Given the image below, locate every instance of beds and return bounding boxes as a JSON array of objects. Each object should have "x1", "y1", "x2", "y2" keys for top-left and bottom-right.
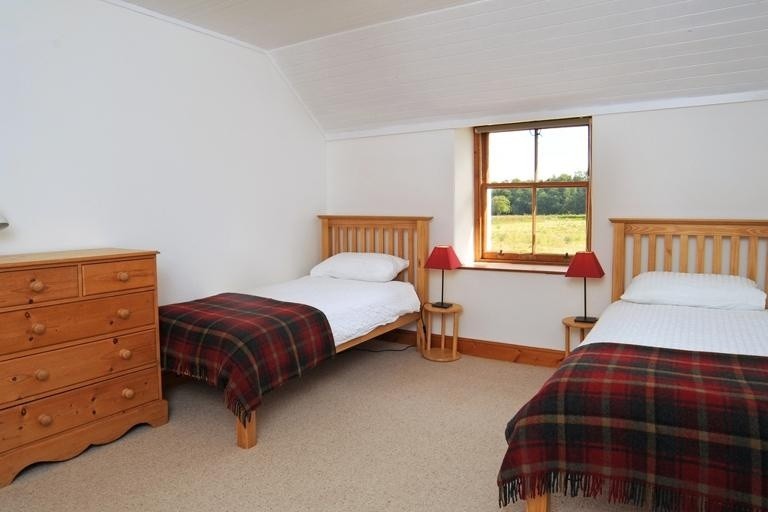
[
  {"x1": 157, "y1": 213, "x2": 433, "y2": 451},
  {"x1": 495, "y1": 217, "x2": 767, "y2": 512}
]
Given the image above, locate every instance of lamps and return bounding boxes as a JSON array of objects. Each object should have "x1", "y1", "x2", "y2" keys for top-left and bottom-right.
[
  {"x1": 422, "y1": 245, "x2": 462, "y2": 309},
  {"x1": 564, "y1": 251, "x2": 605, "y2": 324}
]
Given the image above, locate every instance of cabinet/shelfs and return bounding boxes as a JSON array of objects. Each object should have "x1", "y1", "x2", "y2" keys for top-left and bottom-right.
[{"x1": 0, "y1": 248, "x2": 168, "y2": 490}]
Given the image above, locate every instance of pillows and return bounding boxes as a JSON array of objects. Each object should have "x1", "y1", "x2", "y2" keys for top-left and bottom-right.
[
  {"x1": 309, "y1": 251, "x2": 410, "y2": 283},
  {"x1": 618, "y1": 270, "x2": 766, "y2": 313}
]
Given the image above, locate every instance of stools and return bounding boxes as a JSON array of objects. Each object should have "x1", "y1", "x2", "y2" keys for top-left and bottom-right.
[
  {"x1": 422, "y1": 302, "x2": 464, "y2": 363},
  {"x1": 562, "y1": 317, "x2": 599, "y2": 359}
]
